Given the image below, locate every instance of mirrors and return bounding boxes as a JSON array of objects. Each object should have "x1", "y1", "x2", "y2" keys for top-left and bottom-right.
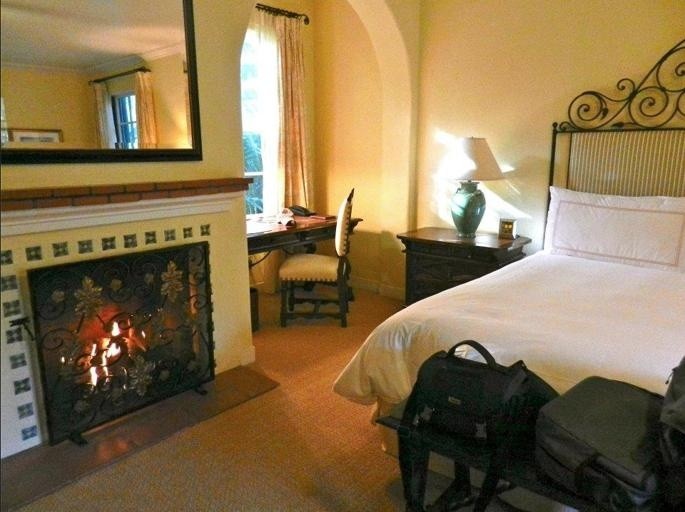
[{"x1": 0, "y1": 0, "x2": 202, "y2": 161}]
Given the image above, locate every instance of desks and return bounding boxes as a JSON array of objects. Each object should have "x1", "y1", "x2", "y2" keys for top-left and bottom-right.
[{"x1": 245, "y1": 212, "x2": 364, "y2": 303}]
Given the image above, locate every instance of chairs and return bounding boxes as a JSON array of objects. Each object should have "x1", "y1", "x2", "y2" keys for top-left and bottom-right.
[{"x1": 279, "y1": 187, "x2": 355, "y2": 326}]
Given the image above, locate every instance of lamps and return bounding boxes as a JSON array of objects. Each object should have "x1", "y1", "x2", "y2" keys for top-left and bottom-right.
[{"x1": 441, "y1": 136, "x2": 505, "y2": 238}]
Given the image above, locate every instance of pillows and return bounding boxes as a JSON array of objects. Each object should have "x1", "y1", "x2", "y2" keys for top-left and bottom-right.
[{"x1": 543, "y1": 186, "x2": 685, "y2": 272}]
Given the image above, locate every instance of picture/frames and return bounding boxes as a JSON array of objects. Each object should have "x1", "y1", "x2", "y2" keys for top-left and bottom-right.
[
  {"x1": 498, "y1": 218, "x2": 517, "y2": 238},
  {"x1": 8, "y1": 128, "x2": 63, "y2": 144}
]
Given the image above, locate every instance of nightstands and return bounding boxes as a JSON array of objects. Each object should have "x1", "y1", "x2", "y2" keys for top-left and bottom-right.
[{"x1": 397, "y1": 227, "x2": 532, "y2": 305}]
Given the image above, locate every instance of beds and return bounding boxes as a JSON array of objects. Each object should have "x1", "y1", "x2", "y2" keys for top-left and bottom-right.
[{"x1": 332, "y1": 42, "x2": 685, "y2": 512}]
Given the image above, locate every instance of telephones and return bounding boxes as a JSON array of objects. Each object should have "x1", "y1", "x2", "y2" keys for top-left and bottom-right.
[{"x1": 290, "y1": 205, "x2": 314, "y2": 216}]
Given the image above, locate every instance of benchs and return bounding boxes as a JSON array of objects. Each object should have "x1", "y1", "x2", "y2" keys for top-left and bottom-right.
[{"x1": 376, "y1": 397, "x2": 620, "y2": 511}]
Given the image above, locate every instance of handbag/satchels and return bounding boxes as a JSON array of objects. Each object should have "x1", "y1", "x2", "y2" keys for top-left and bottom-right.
[
  {"x1": 535, "y1": 357, "x2": 685, "y2": 512},
  {"x1": 403, "y1": 340, "x2": 560, "y2": 457}
]
